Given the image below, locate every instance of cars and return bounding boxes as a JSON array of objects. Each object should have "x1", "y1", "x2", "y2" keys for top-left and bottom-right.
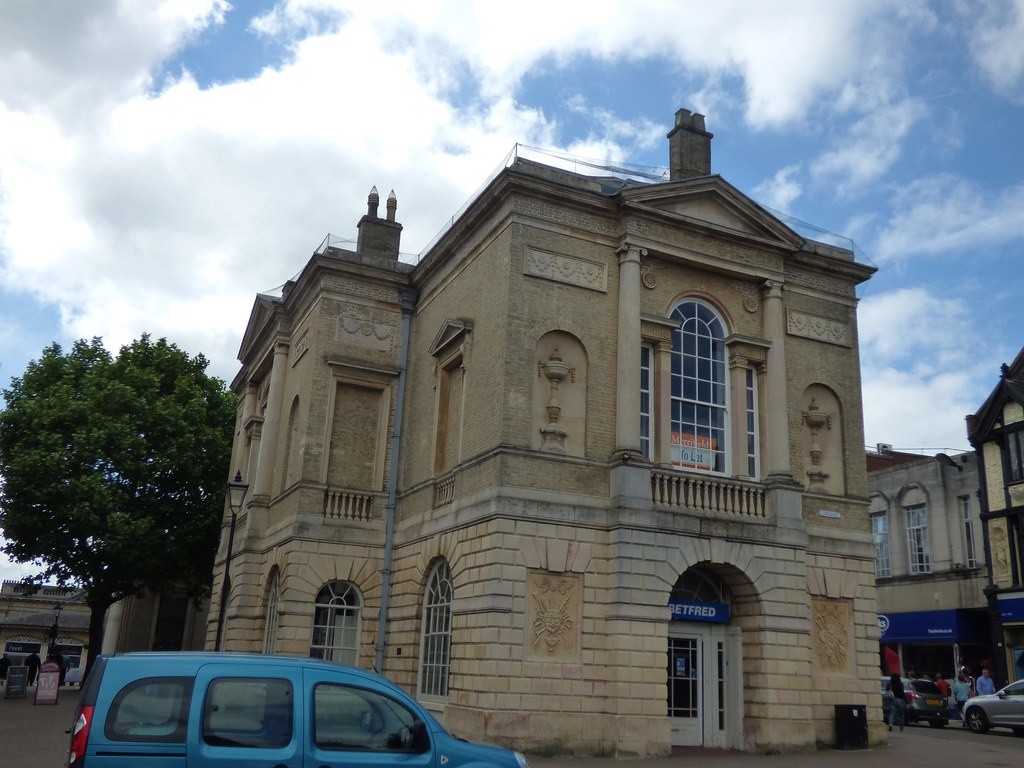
[
  {"x1": 881, "y1": 676, "x2": 891, "y2": 699},
  {"x1": 883, "y1": 678, "x2": 949, "y2": 728},
  {"x1": 960, "y1": 679, "x2": 1024, "y2": 733}
]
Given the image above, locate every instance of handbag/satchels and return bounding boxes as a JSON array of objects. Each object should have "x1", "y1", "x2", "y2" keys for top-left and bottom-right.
[
  {"x1": 884, "y1": 682, "x2": 895, "y2": 700},
  {"x1": 948, "y1": 688, "x2": 951, "y2": 697}
]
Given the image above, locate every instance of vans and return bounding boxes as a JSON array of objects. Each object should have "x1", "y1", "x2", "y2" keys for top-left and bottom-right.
[{"x1": 66, "y1": 651, "x2": 531, "y2": 768}]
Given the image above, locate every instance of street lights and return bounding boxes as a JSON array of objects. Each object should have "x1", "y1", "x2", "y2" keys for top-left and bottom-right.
[
  {"x1": 50, "y1": 603, "x2": 63, "y2": 660},
  {"x1": 214, "y1": 468, "x2": 250, "y2": 651}
]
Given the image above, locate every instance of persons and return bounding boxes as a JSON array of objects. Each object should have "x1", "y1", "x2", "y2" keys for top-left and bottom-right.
[
  {"x1": 976, "y1": 669, "x2": 995, "y2": 695},
  {"x1": 0, "y1": 652, "x2": 11, "y2": 686},
  {"x1": 24, "y1": 651, "x2": 41, "y2": 686},
  {"x1": 952, "y1": 674, "x2": 970, "y2": 727},
  {"x1": 886, "y1": 672, "x2": 908, "y2": 733},
  {"x1": 934, "y1": 674, "x2": 950, "y2": 706},
  {"x1": 908, "y1": 671, "x2": 916, "y2": 680},
  {"x1": 59, "y1": 652, "x2": 70, "y2": 686}
]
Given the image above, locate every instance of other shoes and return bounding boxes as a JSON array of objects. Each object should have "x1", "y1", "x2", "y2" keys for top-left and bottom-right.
[
  {"x1": 900, "y1": 725, "x2": 903, "y2": 732},
  {"x1": 963, "y1": 721, "x2": 966, "y2": 727},
  {"x1": 889, "y1": 725, "x2": 892, "y2": 731}
]
